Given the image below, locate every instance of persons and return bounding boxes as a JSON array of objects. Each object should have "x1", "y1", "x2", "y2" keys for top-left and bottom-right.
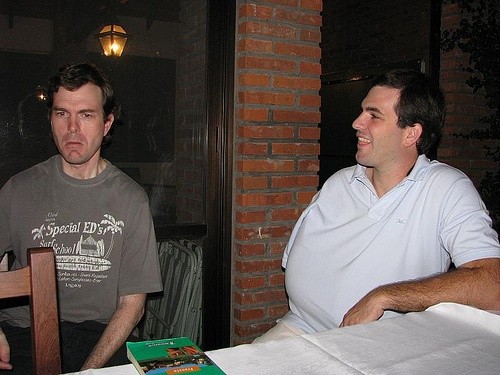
[
  {"x1": 247, "y1": 68, "x2": 500, "y2": 345},
  {"x1": 0, "y1": 61, "x2": 164, "y2": 375}
]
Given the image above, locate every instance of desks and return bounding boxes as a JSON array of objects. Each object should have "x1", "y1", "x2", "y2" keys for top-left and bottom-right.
[{"x1": 63, "y1": 301, "x2": 500, "y2": 375}]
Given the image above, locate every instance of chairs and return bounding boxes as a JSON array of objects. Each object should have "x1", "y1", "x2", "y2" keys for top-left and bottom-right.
[
  {"x1": 0, "y1": 246, "x2": 61, "y2": 375},
  {"x1": 139, "y1": 237, "x2": 204, "y2": 347}
]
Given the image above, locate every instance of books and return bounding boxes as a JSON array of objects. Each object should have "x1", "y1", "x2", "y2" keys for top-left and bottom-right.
[{"x1": 125, "y1": 336, "x2": 227, "y2": 374}]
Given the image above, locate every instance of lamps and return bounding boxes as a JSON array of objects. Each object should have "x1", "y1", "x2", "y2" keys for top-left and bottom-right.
[{"x1": 94, "y1": 0, "x2": 130, "y2": 75}]
